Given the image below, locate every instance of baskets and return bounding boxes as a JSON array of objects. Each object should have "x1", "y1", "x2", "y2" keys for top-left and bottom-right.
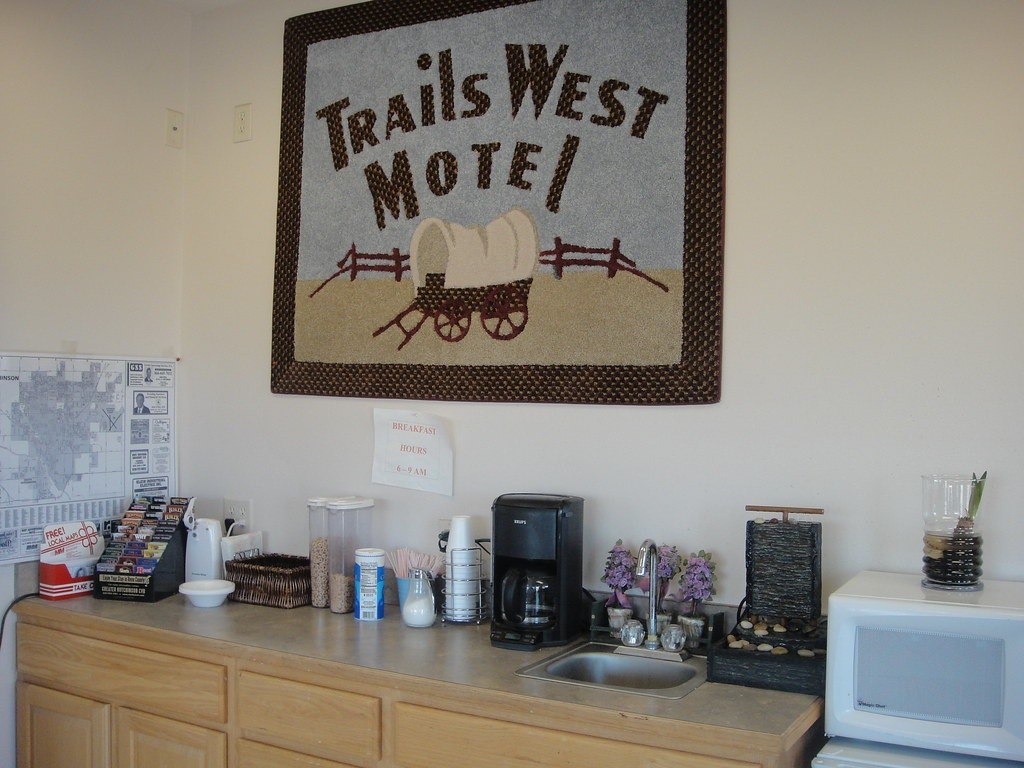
[{"x1": 225, "y1": 553, "x2": 311, "y2": 609}]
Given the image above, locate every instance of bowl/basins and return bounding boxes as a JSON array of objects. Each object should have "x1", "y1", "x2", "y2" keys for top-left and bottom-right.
[{"x1": 179, "y1": 580, "x2": 235, "y2": 607}]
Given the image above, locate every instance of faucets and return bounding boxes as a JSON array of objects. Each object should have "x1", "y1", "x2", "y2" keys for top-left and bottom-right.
[{"x1": 636, "y1": 538, "x2": 661, "y2": 650}]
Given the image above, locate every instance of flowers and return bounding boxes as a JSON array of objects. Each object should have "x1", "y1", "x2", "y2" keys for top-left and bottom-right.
[
  {"x1": 678, "y1": 549, "x2": 717, "y2": 601},
  {"x1": 643, "y1": 543, "x2": 681, "y2": 584},
  {"x1": 599, "y1": 538, "x2": 637, "y2": 609}
]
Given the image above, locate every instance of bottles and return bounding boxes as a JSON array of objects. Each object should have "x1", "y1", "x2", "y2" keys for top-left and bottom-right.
[{"x1": 305, "y1": 494, "x2": 374, "y2": 613}]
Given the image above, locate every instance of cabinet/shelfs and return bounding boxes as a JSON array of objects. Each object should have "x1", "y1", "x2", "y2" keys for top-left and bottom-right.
[{"x1": 11, "y1": 618, "x2": 822, "y2": 768}]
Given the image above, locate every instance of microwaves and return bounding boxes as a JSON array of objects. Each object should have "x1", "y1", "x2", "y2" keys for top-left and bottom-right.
[{"x1": 825, "y1": 572, "x2": 1024, "y2": 763}]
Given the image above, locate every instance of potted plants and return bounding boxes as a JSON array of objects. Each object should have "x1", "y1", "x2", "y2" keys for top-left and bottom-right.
[{"x1": 921, "y1": 470, "x2": 988, "y2": 594}]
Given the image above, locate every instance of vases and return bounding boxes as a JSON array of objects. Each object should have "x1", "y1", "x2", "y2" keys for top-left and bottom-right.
[
  {"x1": 607, "y1": 586, "x2": 634, "y2": 640},
  {"x1": 678, "y1": 597, "x2": 708, "y2": 648},
  {"x1": 645, "y1": 578, "x2": 671, "y2": 635}
]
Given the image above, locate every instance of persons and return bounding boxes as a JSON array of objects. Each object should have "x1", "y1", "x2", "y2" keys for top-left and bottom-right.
[
  {"x1": 145, "y1": 368, "x2": 153, "y2": 382},
  {"x1": 134, "y1": 393, "x2": 150, "y2": 414}
]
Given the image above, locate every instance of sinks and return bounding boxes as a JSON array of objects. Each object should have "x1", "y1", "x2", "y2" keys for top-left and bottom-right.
[{"x1": 514, "y1": 639, "x2": 708, "y2": 699}]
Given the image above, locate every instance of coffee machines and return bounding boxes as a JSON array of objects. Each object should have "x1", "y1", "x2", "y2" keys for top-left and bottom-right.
[{"x1": 489, "y1": 492, "x2": 584, "y2": 651}]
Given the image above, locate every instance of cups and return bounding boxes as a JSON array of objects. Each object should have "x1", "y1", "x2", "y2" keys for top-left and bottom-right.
[{"x1": 445, "y1": 515, "x2": 478, "y2": 622}]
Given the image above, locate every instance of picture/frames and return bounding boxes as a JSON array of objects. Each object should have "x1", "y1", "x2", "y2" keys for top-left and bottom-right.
[{"x1": 271, "y1": 0, "x2": 727, "y2": 405}]
